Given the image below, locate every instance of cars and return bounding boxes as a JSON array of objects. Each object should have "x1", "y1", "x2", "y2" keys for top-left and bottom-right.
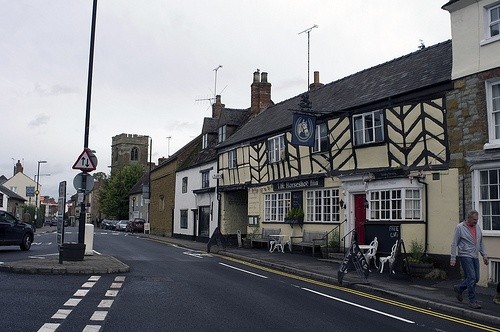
[
  {"x1": 45, "y1": 220, "x2": 57, "y2": 227},
  {"x1": 99, "y1": 218, "x2": 145, "y2": 233},
  {"x1": 0, "y1": 210, "x2": 34, "y2": 251}
]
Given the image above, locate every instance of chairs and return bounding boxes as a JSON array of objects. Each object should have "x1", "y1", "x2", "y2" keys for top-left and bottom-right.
[
  {"x1": 379, "y1": 239, "x2": 399, "y2": 277},
  {"x1": 362, "y1": 237, "x2": 379, "y2": 272}
]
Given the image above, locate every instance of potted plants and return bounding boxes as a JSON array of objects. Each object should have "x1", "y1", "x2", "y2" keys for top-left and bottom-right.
[
  {"x1": 284, "y1": 207, "x2": 304, "y2": 226},
  {"x1": 403, "y1": 237, "x2": 434, "y2": 281},
  {"x1": 320, "y1": 228, "x2": 341, "y2": 259},
  {"x1": 242, "y1": 233, "x2": 254, "y2": 249}
]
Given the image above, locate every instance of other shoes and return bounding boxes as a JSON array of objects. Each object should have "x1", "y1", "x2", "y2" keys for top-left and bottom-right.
[
  {"x1": 454, "y1": 285, "x2": 463, "y2": 301},
  {"x1": 468, "y1": 301, "x2": 482, "y2": 309}
]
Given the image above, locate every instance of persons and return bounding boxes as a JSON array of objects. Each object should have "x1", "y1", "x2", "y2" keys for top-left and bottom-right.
[
  {"x1": 96, "y1": 216, "x2": 101, "y2": 227},
  {"x1": 449, "y1": 208, "x2": 489, "y2": 310},
  {"x1": 92, "y1": 218, "x2": 94, "y2": 224},
  {"x1": 23, "y1": 210, "x2": 31, "y2": 223}
]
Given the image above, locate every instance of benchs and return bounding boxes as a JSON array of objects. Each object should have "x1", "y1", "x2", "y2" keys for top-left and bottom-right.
[
  {"x1": 251, "y1": 228, "x2": 281, "y2": 250},
  {"x1": 290, "y1": 229, "x2": 328, "y2": 257}
]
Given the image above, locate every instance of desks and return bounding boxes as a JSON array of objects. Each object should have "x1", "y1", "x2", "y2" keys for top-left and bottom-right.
[
  {"x1": 358, "y1": 245, "x2": 374, "y2": 254},
  {"x1": 269, "y1": 234, "x2": 289, "y2": 254}
]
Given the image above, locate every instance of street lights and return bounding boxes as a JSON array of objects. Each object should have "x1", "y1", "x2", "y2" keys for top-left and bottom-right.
[
  {"x1": 34, "y1": 161, "x2": 47, "y2": 232},
  {"x1": 34, "y1": 174, "x2": 50, "y2": 182}
]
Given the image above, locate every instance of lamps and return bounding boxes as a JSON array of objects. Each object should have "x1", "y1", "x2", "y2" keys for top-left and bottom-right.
[
  {"x1": 212, "y1": 173, "x2": 223, "y2": 179},
  {"x1": 159, "y1": 193, "x2": 164, "y2": 200},
  {"x1": 339, "y1": 199, "x2": 346, "y2": 210}
]
